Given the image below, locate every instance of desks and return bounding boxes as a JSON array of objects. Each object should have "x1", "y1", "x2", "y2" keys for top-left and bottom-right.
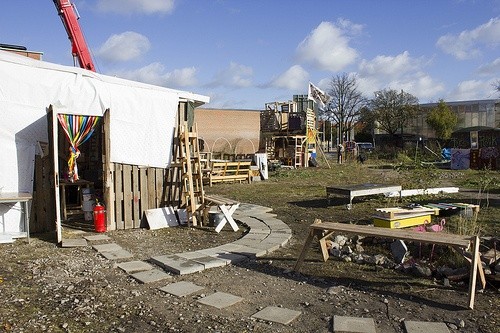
[
  {"x1": 292, "y1": 220, "x2": 486, "y2": 309},
  {"x1": 59, "y1": 178, "x2": 94, "y2": 222},
  {"x1": 326, "y1": 183, "x2": 402, "y2": 210},
  {"x1": 0, "y1": 192, "x2": 33, "y2": 245}
]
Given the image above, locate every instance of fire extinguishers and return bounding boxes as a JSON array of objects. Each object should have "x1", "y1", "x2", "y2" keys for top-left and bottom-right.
[{"x1": 92, "y1": 199, "x2": 106, "y2": 233}]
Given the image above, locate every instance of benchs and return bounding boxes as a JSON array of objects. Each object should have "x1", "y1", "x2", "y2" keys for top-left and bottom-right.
[
  {"x1": 203, "y1": 161, "x2": 251, "y2": 187},
  {"x1": 201, "y1": 195, "x2": 240, "y2": 234}
]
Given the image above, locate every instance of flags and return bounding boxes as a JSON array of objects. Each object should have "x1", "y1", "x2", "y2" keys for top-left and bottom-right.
[{"x1": 307, "y1": 81, "x2": 332, "y2": 109}]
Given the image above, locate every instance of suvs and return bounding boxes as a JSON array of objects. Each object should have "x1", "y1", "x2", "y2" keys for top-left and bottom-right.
[{"x1": 355, "y1": 142, "x2": 377, "y2": 159}]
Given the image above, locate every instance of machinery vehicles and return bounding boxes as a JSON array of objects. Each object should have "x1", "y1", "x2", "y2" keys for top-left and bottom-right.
[{"x1": 51, "y1": 0, "x2": 101, "y2": 73}]
